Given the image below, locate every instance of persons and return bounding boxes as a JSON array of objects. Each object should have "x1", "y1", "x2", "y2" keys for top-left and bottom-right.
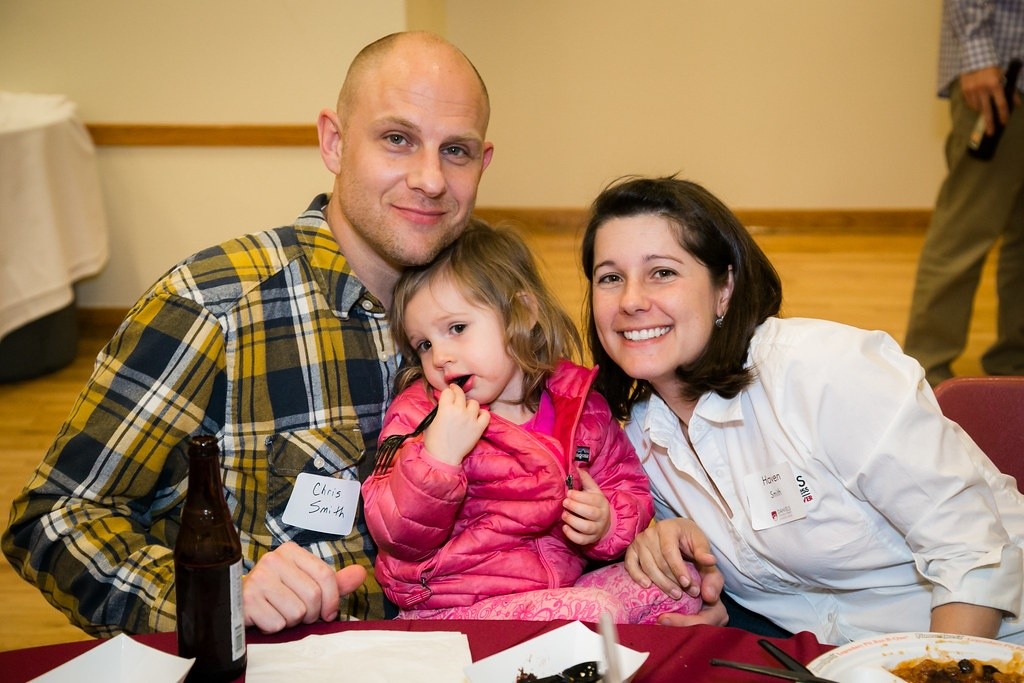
[
  {"x1": 581, "y1": 177, "x2": 1024, "y2": 645},
  {"x1": 903, "y1": 0, "x2": 1024, "y2": 386},
  {"x1": 1, "y1": 31, "x2": 728, "y2": 639},
  {"x1": 361, "y1": 221, "x2": 703, "y2": 625}
]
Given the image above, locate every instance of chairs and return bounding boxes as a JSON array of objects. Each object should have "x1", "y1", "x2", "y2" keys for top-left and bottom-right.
[{"x1": 933, "y1": 376, "x2": 1024, "y2": 498}]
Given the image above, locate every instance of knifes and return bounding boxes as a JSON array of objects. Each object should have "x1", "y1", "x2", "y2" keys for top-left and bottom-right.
[{"x1": 709, "y1": 658, "x2": 839, "y2": 683}]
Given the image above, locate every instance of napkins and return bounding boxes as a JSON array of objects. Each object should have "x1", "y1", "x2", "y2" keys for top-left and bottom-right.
[{"x1": 245, "y1": 629, "x2": 472, "y2": 683}]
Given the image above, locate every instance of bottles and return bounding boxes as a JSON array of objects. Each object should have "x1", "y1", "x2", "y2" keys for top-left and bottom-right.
[{"x1": 172, "y1": 433, "x2": 247, "y2": 683}]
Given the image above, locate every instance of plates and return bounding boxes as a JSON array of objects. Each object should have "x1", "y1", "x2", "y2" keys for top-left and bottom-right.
[{"x1": 805, "y1": 631, "x2": 1024, "y2": 683}]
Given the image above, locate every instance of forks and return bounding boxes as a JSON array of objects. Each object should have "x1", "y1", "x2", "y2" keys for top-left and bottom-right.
[{"x1": 372, "y1": 377, "x2": 471, "y2": 476}]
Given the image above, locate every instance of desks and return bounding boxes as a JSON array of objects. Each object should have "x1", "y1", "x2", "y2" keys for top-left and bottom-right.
[
  {"x1": 0, "y1": 621, "x2": 837, "y2": 683},
  {"x1": 0, "y1": 89, "x2": 111, "y2": 382}
]
